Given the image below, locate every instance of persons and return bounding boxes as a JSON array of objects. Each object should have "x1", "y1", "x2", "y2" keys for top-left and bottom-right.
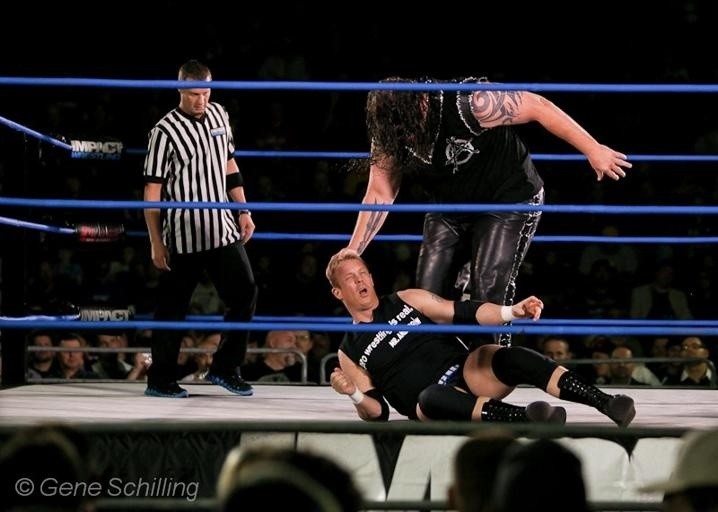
[
  {"x1": 214, "y1": 444, "x2": 364, "y2": 511},
  {"x1": 324, "y1": 247, "x2": 636, "y2": 442},
  {"x1": 3, "y1": 435, "x2": 92, "y2": 511},
  {"x1": 331, "y1": 77, "x2": 633, "y2": 349},
  {"x1": 495, "y1": 438, "x2": 587, "y2": 512},
  {"x1": 633, "y1": 427, "x2": 718, "y2": 510},
  {"x1": 19, "y1": 221, "x2": 715, "y2": 389},
  {"x1": 445, "y1": 436, "x2": 524, "y2": 510},
  {"x1": 140, "y1": 58, "x2": 257, "y2": 400}
]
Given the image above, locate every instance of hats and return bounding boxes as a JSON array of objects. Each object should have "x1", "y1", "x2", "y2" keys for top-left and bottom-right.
[{"x1": 635, "y1": 429, "x2": 718, "y2": 493}]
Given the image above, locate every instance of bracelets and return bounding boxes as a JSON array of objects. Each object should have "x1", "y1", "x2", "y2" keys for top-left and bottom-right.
[
  {"x1": 350, "y1": 385, "x2": 364, "y2": 405},
  {"x1": 238, "y1": 209, "x2": 250, "y2": 216}
]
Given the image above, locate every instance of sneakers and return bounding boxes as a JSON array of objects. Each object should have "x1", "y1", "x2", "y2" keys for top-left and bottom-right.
[
  {"x1": 204, "y1": 364, "x2": 253, "y2": 396},
  {"x1": 144, "y1": 380, "x2": 188, "y2": 398}
]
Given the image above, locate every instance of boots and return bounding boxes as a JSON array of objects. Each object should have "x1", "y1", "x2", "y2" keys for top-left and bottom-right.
[
  {"x1": 480, "y1": 398, "x2": 566, "y2": 424},
  {"x1": 559, "y1": 370, "x2": 635, "y2": 428}
]
